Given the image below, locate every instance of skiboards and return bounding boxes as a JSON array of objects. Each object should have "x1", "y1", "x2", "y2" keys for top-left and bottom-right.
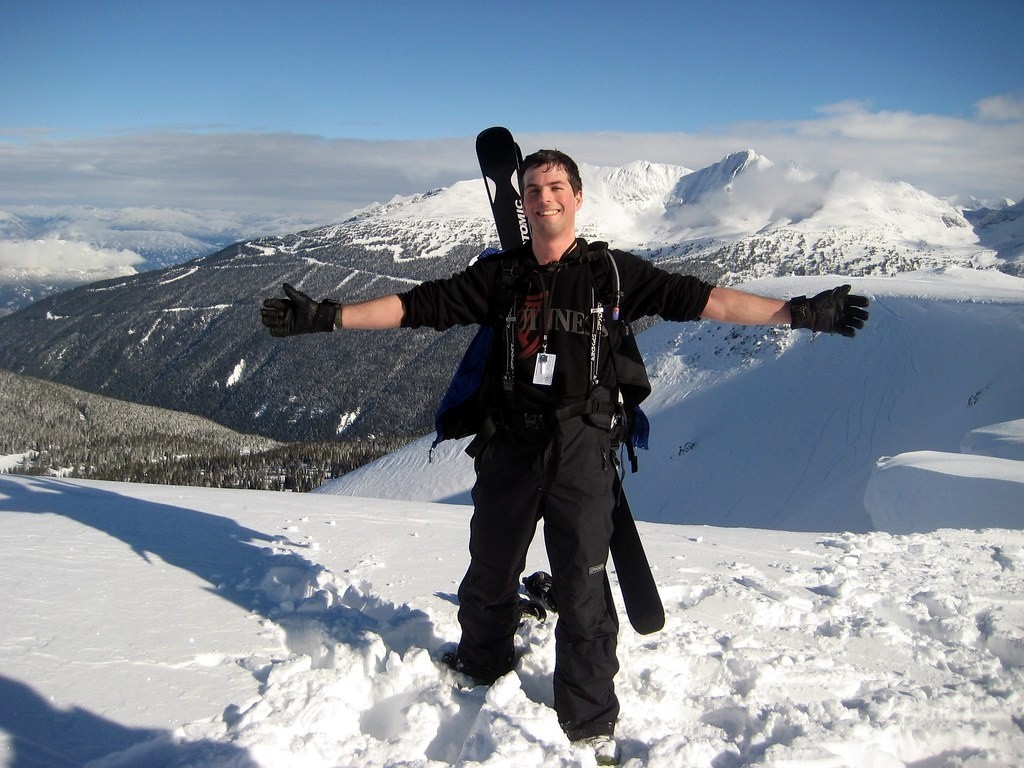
[{"x1": 475, "y1": 127, "x2": 667, "y2": 635}]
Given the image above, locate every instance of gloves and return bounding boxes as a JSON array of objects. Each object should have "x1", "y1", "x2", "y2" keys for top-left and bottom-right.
[
  {"x1": 790, "y1": 284, "x2": 870, "y2": 338},
  {"x1": 260, "y1": 282, "x2": 342, "y2": 337}
]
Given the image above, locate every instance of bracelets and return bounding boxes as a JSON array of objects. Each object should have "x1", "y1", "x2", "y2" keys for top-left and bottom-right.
[{"x1": 333, "y1": 304, "x2": 343, "y2": 330}]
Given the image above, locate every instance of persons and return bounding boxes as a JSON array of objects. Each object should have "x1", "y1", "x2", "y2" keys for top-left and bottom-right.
[{"x1": 261, "y1": 149, "x2": 869, "y2": 765}]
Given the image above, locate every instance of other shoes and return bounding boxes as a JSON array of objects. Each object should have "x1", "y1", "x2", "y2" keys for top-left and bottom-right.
[{"x1": 584, "y1": 735, "x2": 621, "y2": 766}]
